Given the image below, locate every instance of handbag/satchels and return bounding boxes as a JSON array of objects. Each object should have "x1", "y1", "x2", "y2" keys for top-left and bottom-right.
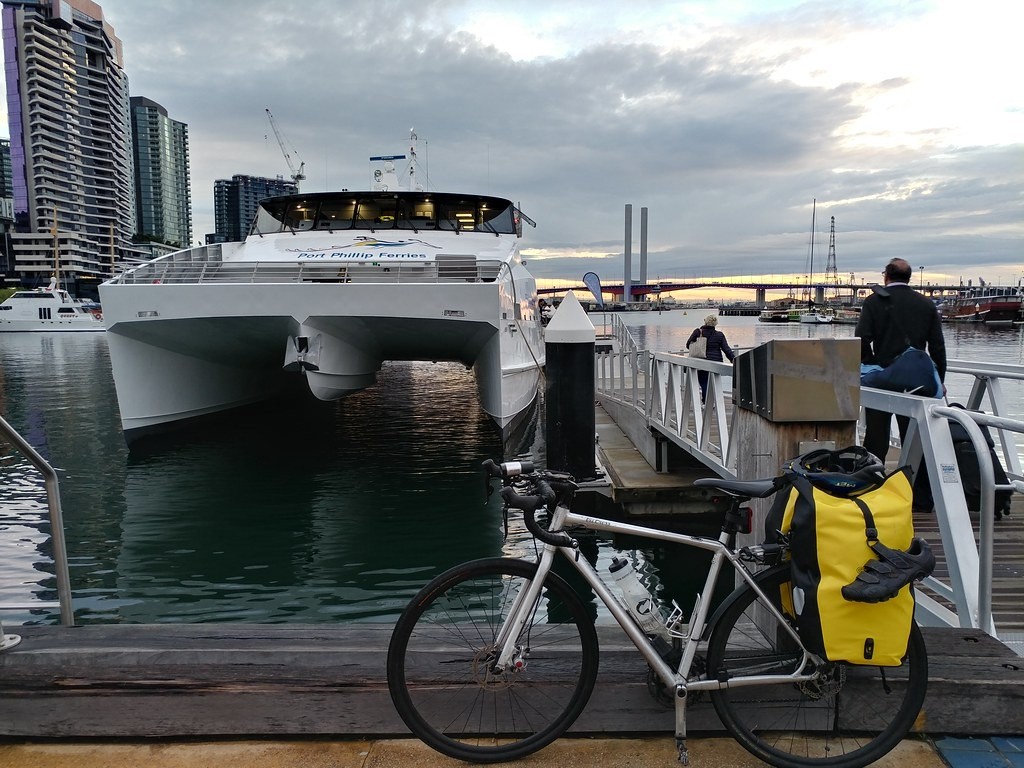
[
  {"x1": 764, "y1": 465, "x2": 917, "y2": 668},
  {"x1": 860, "y1": 347, "x2": 945, "y2": 400},
  {"x1": 689, "y1": 328, "x2": 707, "y2": 358}
]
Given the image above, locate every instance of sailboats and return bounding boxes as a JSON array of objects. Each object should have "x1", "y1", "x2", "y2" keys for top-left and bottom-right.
[
  {"x1": 0, "y1": 204, "x2": 107, "y2": 334},
  {"x1": 717, "y1": 194, "x2": 862, "y2": 327}
]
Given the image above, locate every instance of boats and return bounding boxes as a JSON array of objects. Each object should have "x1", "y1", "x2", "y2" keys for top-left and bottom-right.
[
  {"x1": 936, "y1": 294, "x2": 1024, "y2": 327},
  {"x1": 553, "y1": 296, "x2": 718, "y2": 315},
  {"x1": 94, "y1": 125, "x2": 545, "y2": 465}
]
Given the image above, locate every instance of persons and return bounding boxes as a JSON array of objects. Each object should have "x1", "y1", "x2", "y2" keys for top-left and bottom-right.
[
  {"x1": 854, "y1": 259, "x2": 947, "y2": 468},
  {"x1": 685, "y1": 315, "x2": 735, "y2": 411}
]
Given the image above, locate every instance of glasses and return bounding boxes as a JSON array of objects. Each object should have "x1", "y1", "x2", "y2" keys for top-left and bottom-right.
[{"x1": 882, "y1": 271, "x2": 887, "y2": 277}]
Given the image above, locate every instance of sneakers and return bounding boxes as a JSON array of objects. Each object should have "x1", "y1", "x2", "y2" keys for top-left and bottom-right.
[{"x1": 841, "y1": 537, "x2": 937, "y2": 604}]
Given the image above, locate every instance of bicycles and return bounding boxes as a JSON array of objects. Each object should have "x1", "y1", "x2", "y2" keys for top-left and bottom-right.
[{"x1": 383, "y1": 458, "x2": 931, "y2": 768}]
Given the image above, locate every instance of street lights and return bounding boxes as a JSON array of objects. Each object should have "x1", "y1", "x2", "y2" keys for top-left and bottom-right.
[{"x1": 918, "y1": 265, "x2": 924, "y2": 294}]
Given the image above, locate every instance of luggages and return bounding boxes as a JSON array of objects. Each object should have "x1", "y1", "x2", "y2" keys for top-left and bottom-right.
[{"x1": 943, "y1": 391, "x2": 1013, "y2": 522}]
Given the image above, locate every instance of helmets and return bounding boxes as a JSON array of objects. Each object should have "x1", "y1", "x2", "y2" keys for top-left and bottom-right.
[{"x1": 790, "y1": 446, "x2": 884, "y2": 494}]
